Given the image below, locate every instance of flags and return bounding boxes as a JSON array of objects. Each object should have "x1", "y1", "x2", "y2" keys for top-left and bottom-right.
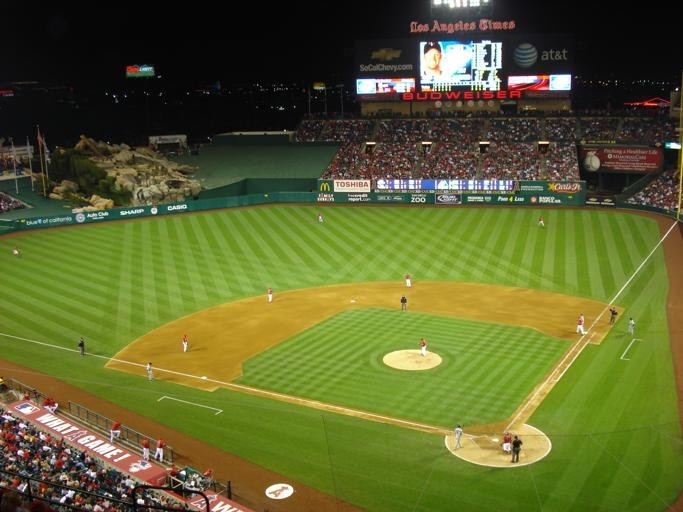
[{"x1": 11, "y1": 128, "x2": 52, "y2": 165}]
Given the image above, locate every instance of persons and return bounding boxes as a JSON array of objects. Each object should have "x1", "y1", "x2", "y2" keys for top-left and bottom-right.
[
  {"x1": 292, "y1": 109, "x2": 683, "y2": 213},
  {"x1": 454, "y1": 424, "x2": 523, "y2": 462},
  {"x1": 406, "y1": 272, "x2": 411, "y2": 288},
  {"x1": 147, "y1": 363, "x2": 154, "y2": 382},
  {"x1": 420, "y1": 41, "x2": 443, "y2": 83},
  {"x1": 183, "y1": 335, "x2": 188, "y2": 352},
  {"x1": 418, "y1": 337, "x2": 427, "y2": 357},
  {"x1": 576, "y1": 307, "x2": 635, "y2": 335},
  {"x1": 401, "y1": 296, "x2": 407, "y2": 310},
  {"x1": 78, "y1": 337, "x2": 84, "y2": 356},
  {"x1": 0, "y1": 376, "x2": 215, "y2": 512},
  {"x1": 268, "y1": 288, "x2": 273, "y2": 303}
]
